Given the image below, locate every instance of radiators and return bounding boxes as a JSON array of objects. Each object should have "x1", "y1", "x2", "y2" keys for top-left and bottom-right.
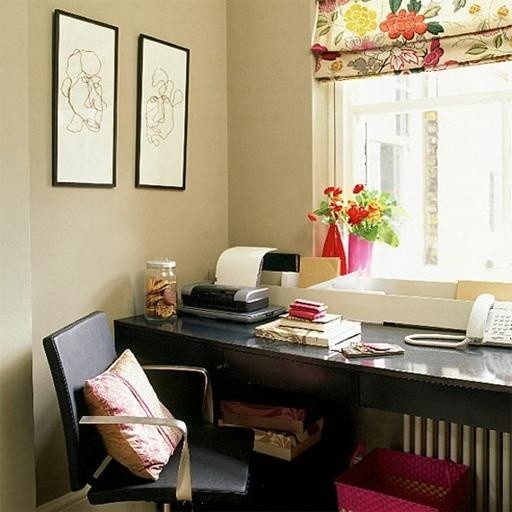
[{"x1": 402, "y1": 414, "x2": 512, "y2": 512}]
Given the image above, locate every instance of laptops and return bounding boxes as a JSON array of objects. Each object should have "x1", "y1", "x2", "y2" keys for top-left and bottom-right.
[{"x1": 176, "y1": 304, "x2": 286, "y2": 324}]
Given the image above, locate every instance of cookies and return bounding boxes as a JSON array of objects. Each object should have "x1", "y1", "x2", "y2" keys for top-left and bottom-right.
[{"x1": 145, "y1": 277, "x2": 177, "y2": 321}]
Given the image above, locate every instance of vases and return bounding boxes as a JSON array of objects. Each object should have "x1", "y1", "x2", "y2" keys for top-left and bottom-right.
[
  {"x1": 321, "y1": 224, "x2": 346, "y2": 275},
  {"x1": 348, "y1": 234, "x2": 373, "y2": 274}
]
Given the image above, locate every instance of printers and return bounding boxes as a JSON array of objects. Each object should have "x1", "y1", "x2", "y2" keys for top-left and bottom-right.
[{"x1": 181, "y1": 258, "x2": 269, "y2": 314}]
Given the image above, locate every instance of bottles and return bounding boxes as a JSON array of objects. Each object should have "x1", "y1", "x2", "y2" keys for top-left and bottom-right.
[{"x1": 144, "y1": 257, "x2": 177, "y2": 324}]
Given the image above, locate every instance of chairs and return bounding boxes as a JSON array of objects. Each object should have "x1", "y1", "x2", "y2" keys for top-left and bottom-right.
[{"x1": 42, "y1": 310, "x2": 255, "y2": 512}]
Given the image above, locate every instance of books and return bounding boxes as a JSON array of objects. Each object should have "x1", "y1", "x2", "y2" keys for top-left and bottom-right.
[{"x1": 255, "y1": 312, "x2": 361, "y2": 348}]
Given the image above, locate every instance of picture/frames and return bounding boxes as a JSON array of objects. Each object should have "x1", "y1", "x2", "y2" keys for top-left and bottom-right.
[
  {"x1": 53, "y1": 9, "x2": 118, "y2": 188},
  {"x1": 135, "y1": 34, "x2": 190, "y2": 191}
]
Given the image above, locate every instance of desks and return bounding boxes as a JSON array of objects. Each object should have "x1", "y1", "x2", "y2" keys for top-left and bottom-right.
[{"x1": 114, "y1": 308, "x2": 512, "y2": 434}]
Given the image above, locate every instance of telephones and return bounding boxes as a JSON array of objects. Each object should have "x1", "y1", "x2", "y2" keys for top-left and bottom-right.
[{"x1": 467, "y1": 294, "x2": 512, "y2": 348}]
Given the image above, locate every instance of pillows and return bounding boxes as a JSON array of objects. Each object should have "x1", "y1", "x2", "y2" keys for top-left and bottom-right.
[{"x1": 82, "y1": 349, "x2": 183, "y2": 481}]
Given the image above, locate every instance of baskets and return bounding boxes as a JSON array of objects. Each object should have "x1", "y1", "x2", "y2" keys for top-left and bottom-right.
[{"x1": 334, "y1": 447, "x2": 471, "y2": 512}]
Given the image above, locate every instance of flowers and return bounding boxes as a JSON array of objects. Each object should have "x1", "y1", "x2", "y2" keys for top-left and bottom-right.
[
  {"x1": 337, "y1": 183, "x2": 409, "y2": 248},
  {"x1": 305, "y1": 186, "x2": 348, "y2": 224}
]
